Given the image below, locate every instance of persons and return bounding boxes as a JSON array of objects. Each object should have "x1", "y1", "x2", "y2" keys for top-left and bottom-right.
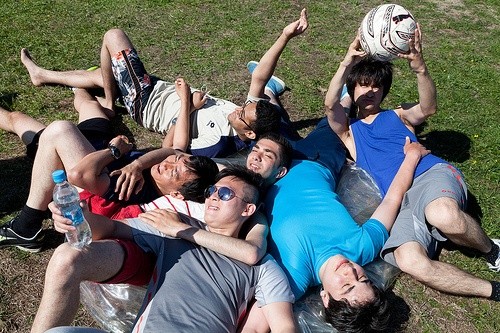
[
  {"x1": 324, "y1": 22, "x2": 500, "y2": 303},
  {"x1": 0, "y1": 8, "x2": 431, "y2": 333}
]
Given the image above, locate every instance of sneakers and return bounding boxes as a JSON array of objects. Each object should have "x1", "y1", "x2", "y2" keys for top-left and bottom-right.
[
  {"x1": 485, "y1": 238, "x2": 500, "y2": 277},
  {"x1": 244, "y1": 59, "x2": 286, "y2": 95},
  {"x1": 1, "y1": 218, "x2": 45, "y2": 254}
]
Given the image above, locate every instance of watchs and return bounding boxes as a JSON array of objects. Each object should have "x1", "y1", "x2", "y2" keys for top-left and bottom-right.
[{"x1": 108, "y1": 144, "x2": 121, "y2": 159}]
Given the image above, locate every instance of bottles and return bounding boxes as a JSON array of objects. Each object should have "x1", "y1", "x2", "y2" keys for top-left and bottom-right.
[{"x1": 52, "y1": 170, "x2": 92, "y2": 249}]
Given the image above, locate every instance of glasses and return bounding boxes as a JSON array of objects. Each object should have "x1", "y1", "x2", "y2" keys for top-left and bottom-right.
[
  {"x1": 240, "y1": 99, "x2": 255, "y2": 134},
  {"x1": 202, "y1": 184, "x2": 250, "y2": 206}
]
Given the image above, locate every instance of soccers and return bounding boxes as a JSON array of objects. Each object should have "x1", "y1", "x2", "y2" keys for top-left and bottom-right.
[{"x1": 359, "y1": 4, "x2": 416, "y2": 61}]
